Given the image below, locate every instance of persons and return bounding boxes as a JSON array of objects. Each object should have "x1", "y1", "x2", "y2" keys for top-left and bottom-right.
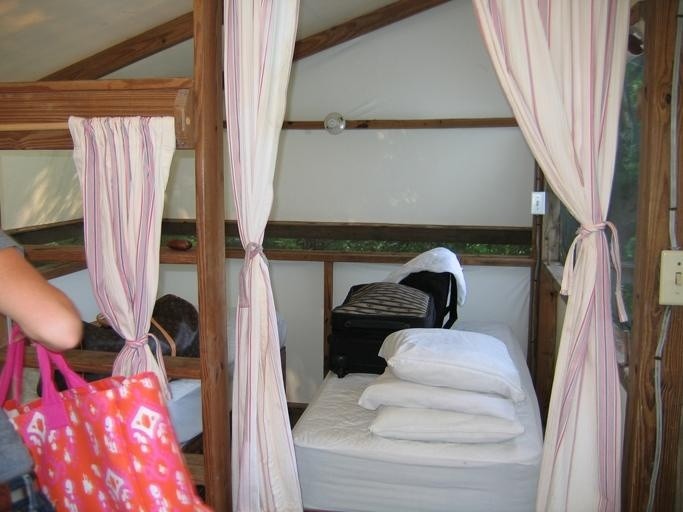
[{"x1": 0, "y1": 231, "x2": 84, "y2": 353}]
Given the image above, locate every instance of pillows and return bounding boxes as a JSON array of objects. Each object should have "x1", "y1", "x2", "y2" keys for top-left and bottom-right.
[
  {"x1": 366, "y1": 406, "x2": 525, "y2": 444},
  {"x1": 378, "y1": 329, "x2": 527, "y2": 403},
  {"x1": 357, "y1": 367, "x2": 516, "y2": 421}
]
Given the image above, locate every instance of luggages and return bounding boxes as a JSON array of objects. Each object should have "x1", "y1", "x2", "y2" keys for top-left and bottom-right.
[{"x1": 327, "y1": 282, "x2": 436, "y2": 379}]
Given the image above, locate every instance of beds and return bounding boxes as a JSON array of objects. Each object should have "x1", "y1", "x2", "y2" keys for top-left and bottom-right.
[
  {"x1": 0, "y1": 309, "x2": 289, "y2": 451},
  {"x1": 292, "y1": 323, "x2": 547, "y2": 512}
]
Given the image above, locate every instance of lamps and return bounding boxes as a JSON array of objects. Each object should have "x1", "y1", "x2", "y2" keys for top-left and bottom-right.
[{"x1": 324, "y1": 113, "x2": 345, "y2": 135}]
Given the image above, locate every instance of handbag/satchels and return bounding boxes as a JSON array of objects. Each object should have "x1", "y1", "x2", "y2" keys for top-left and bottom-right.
[
  {"x1": 85, "y1": 294, "x2": 199, "y2": 355},
  {"x1": 6, "y1": 344, "x2": 203, "y2": 508}
]
